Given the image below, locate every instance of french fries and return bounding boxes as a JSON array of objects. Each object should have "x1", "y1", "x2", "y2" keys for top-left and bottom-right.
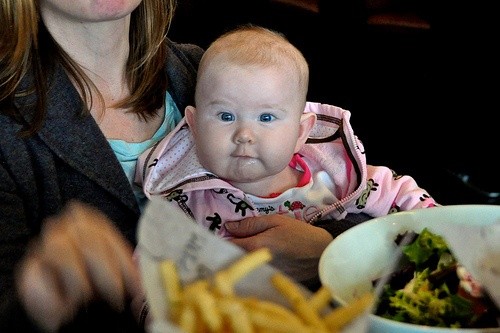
[{"x1": 159, "y1": 246, "x2": 377, "y2": 333}]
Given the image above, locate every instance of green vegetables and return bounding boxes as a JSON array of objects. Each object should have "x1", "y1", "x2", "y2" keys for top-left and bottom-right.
[{"x1": 380, "y1": 228, "x2": 479, "y2": 329}]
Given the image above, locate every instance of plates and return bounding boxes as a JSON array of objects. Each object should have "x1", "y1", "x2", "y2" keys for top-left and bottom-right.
[{"x1": 136, "y1": 197, "x2": 357, "y2": 333}]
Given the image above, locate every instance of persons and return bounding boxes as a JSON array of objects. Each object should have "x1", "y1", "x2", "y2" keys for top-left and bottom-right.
[
  {"x1": 1, "y1": 0, "x2": 381, "y2": 332},
  {"x1": 133, "y1": 27, "x2": 447, "y2": 239}
]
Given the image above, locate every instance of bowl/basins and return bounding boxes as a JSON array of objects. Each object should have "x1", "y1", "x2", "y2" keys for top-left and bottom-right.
[{"x1": 317, "y1": 203, "x2": 500, "y2": 333}]
